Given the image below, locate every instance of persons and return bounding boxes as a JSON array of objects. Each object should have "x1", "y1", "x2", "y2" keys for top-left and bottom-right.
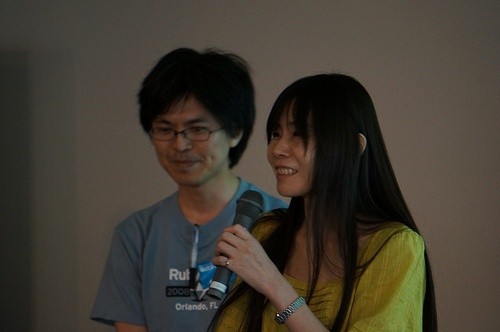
[
  {"x1": 86, "y1": 42, "x2": 290, "y2": 331},
  {"x1": 201, "y1": 72, "x2": 441, "y2": 332}
]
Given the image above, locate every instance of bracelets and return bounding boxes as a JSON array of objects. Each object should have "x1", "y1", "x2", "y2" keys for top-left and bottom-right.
[{"x1": 272, "y1": 289, "x2": 310, "y2": 327}]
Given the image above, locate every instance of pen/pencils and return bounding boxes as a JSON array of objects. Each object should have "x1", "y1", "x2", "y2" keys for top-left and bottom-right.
[{"x1": 189, "y1": 224, "x2": 201, "y2": 289}]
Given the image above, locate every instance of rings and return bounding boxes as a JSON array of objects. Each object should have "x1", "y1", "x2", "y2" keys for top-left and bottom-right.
[{"x1": 224, "y1": 258, "x2": 232, "y2": 269}]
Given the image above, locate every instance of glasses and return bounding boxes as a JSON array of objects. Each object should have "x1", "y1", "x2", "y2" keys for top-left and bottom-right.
[{"x1": 146, "y1": 126, "x2": 224, "y2": 142}]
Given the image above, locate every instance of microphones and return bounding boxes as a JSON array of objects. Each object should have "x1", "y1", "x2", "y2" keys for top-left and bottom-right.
[{"x1": 208, "y1": 189, "x2": 264, "y2": 300}]
